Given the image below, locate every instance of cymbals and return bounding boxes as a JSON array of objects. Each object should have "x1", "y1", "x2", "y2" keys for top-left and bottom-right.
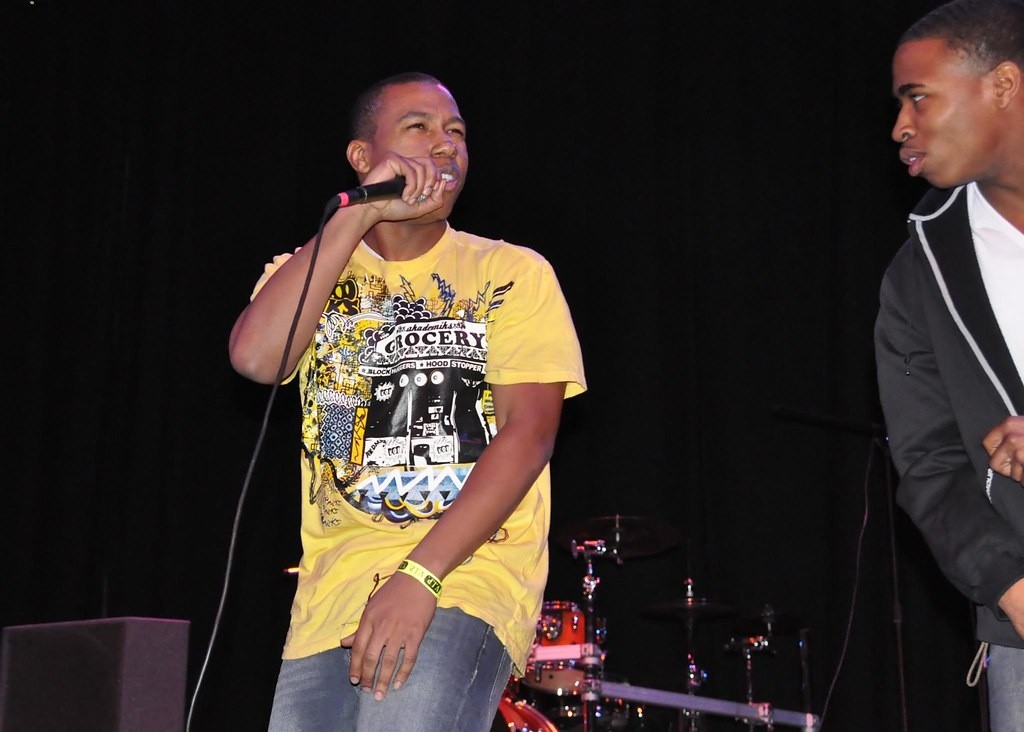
[
  {"x1": 556, "y1": 515, "x2": 682, "y2": 560},
  {"x1": 732, "y1": 614, "x2": 804, "y2": 639},
  {"x1": 641, "y1": 598, "x2": 738, "y2": 621}
]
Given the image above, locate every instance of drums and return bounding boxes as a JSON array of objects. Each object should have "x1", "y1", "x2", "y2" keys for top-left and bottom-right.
[
  {"x1": 514, "y1": 598, "x2": 608, "y2": 697},
  {"x1": 490, "y1": 696, "x2": 559, "y2": 732}
]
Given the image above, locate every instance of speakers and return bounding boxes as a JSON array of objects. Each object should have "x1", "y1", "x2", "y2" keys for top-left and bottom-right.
[{"x1": 0, "y1": 618, "x2": 191, "y2": 732}]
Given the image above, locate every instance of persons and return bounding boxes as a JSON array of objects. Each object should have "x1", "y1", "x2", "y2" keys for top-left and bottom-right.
[
  {"x1": 874, "y1": 0, "x2": 1024, "y2": 732},
  {"x1": 229, "y1": 69, "x2": 588, "y2": 732}
]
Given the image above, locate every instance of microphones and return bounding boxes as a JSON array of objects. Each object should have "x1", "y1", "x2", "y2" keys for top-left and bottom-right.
[{"x1": 324, "y1": 176, "x2": 427, "y2": 215}]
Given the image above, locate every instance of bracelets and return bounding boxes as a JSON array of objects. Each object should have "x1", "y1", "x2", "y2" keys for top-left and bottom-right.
[{"x1": 396, "y1": 560, "x2": 442, "y2": 600}]
